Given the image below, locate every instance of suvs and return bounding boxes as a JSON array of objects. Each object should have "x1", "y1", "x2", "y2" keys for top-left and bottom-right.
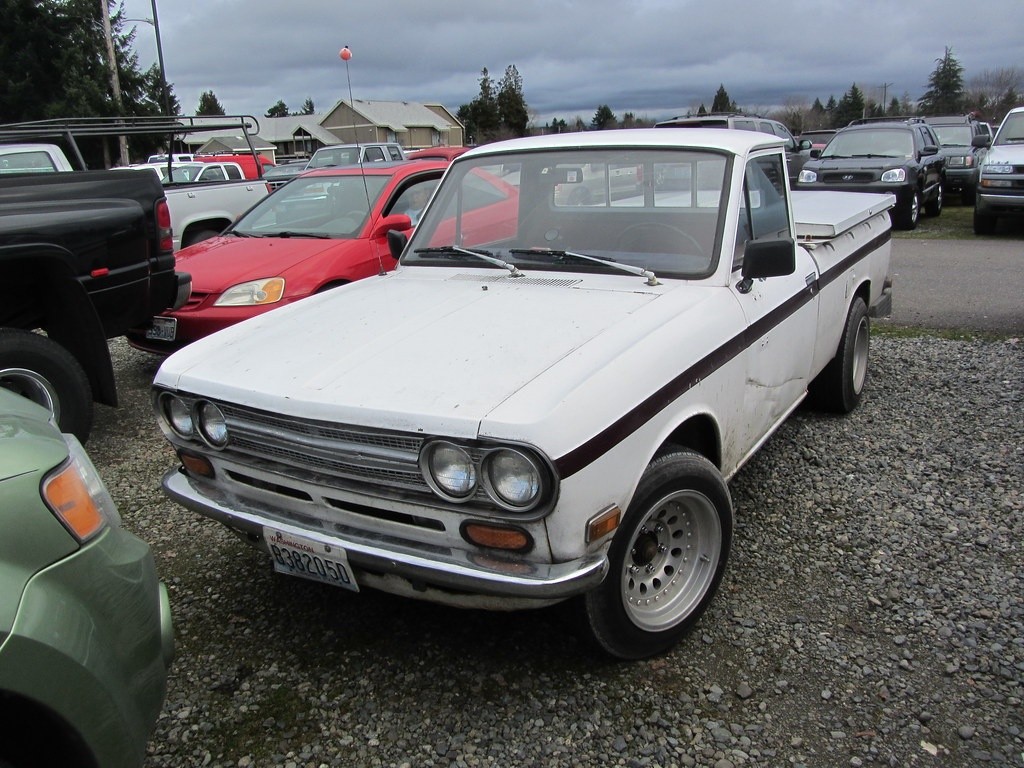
[
  {"x1": 0, "y1": 116, "x2": 472, "y2": 446},
  {"x1": 500, "y1": 106, "x2": 1024, "y2": 235}
]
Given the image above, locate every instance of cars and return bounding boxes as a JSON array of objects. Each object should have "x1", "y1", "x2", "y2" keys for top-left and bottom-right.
[
  {"x1": 0, "y1": 385, "x2": 175, "y2": 768},
  {"x1": 129, "y1": 160, "x2": 519, "y2": 356}
]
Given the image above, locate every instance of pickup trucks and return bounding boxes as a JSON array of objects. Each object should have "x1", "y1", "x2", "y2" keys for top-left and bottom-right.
[{"x1": 147, "y1": 127, "x2": 898, "y2": 661}]
